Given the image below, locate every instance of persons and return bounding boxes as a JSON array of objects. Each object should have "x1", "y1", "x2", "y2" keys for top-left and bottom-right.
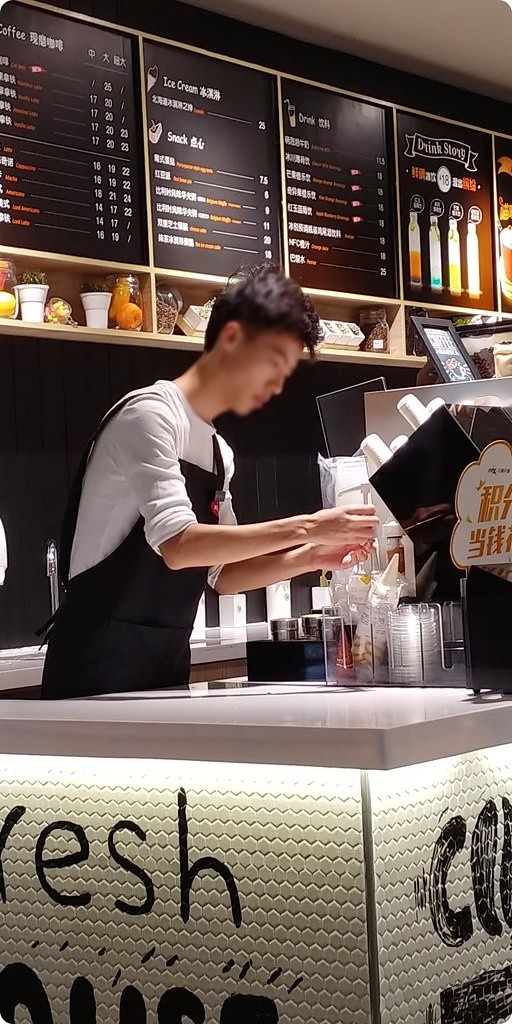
[{"x1": 37, "y1": 261, "x2": 379, "y2": 701}]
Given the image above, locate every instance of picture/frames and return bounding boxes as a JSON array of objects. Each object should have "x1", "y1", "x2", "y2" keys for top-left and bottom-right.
[{"x1": 410, "y1": 316, "x2": 482, "y2": 384}]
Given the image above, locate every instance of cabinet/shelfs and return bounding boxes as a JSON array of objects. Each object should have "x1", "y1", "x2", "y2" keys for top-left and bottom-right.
[{"x1": 0, "y1": 242, "x2": 512, "y2": 370}]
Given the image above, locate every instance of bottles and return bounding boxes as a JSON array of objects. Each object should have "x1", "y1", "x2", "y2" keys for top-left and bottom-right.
[
  {"x1": 382, "y1": 521, "x2": 407, "y2": 580},
  {"x1": 466, "y1": 223, "x2": 480, "y2": 299},
  {"x1": 448, "y1": 219, "x2": 461, "y2": 297},
  {"x1": 429, "y1": 216, "x2": 442, "y2": 290},
  {"x1": 335, "y1": 624, "x2": 354, "y2": 670},
  {"x1": 409, "y1": 212, "x2": 421, "y2": 285}
]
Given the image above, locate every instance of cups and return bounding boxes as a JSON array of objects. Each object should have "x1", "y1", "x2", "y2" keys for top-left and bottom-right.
[
  {"x1": 332, "y1": 395, "x2": 446, "y2": 508},
  {"x1": 500, "y1": 228, "x2": 512, "y2": 282}
]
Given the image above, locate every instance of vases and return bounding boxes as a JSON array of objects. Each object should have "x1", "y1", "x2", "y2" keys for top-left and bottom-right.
[
  {"x1": 13, "y1": 266, "x2": 50, "y2": 323},
  {"x1": 80, "y1": 279, "x2": 113, "y2": 328}
]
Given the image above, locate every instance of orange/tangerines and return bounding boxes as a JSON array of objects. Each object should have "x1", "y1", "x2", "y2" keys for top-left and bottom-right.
[
  {"x1": 0, "y1": 292, "x2": 15, "y2": 315},
  {"x1": 116, "y1": 303, "x2": 142, "y2": 329}
]
